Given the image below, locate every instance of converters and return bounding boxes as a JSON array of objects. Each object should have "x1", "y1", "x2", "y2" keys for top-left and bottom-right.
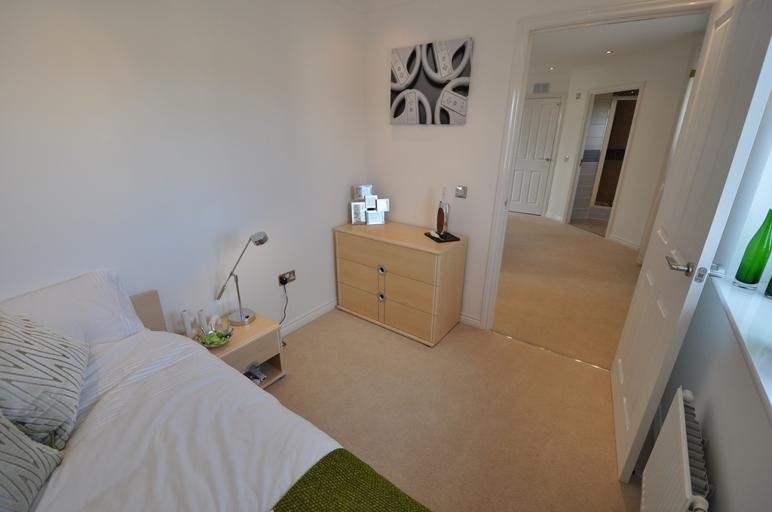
[{"x1": 280, "y1": 277, "x2": 287, "y2": 284}]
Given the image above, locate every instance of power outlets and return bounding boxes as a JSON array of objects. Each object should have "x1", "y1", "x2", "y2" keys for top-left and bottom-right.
[{"x1": 278, "y1": 269, "x2": 295, "y2": 286}]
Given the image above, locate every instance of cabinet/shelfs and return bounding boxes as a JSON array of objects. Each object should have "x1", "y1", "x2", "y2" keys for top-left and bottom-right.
[{"x1": 333, "y1": 221, "x2": 468, "y2": 348}]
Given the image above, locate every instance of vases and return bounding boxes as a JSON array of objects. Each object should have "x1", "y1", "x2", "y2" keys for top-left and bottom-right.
[{"x1": 733, "y1": 208, "x2": 772, "y2": 291}]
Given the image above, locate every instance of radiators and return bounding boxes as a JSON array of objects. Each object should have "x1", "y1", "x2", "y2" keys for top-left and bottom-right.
[{"x1": 640, "y1": 386, "x2": 709, "y2": 512}]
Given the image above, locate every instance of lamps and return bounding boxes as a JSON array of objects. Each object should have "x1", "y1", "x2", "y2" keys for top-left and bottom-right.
[{"x1": 217, "y1": 232, "x2": 268, "y2": 326}]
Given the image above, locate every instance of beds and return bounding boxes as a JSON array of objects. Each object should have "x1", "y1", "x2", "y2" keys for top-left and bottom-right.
[{"x1": 38, "y1": 290, "x2": 428, "y2": 512}]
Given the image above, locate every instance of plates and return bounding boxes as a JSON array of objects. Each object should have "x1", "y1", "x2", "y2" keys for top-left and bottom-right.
[{"x1": 195, "y1": 329, "x2": 229, "y2": 348}]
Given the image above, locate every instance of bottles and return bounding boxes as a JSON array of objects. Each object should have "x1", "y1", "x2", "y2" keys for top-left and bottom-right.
[{"x1": 734, "y1": 209, "x2": 772, "y2": 300}]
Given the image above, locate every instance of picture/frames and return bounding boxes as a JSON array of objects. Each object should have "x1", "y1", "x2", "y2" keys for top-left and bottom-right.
[{"x1": 351, "y1": 185, "x2": 389, "y2": 226}]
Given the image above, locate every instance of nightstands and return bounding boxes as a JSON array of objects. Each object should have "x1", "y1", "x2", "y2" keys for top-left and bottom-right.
[{"x1": 181, "y1": 311, "x2": 286, "y2": 389}]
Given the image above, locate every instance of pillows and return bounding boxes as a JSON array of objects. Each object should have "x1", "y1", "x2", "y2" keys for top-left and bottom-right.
[
  {"x1": 0, "y1": 412, "x2": 64, "y2": 512},
  {"x1": 0, "y1": 271, "x2": 144, "y2": 344},
  {"x1": 0, "y1": 311, "x2": 99, "y2": 449}
]
